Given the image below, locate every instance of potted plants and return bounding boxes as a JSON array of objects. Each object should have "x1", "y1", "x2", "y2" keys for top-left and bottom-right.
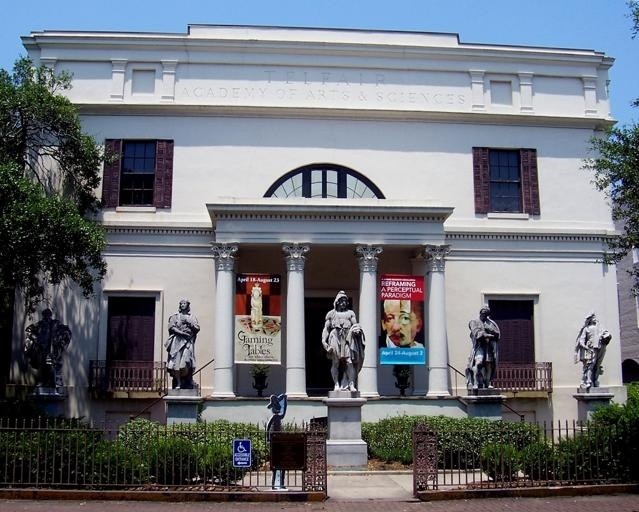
[
  {"x1": 393, "y1": 364, "x2": 413, "y2": 384},
  {"x1": 248, "y1": 364, "x2": 270, "y2": 387}
]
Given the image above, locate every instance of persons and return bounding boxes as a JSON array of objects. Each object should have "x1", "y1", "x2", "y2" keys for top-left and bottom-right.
[
  {"x1": 382, "y1": 299, "x2": 423, "y2": 348},
  {"x1": 574, "y1": 313, "x2": 612, "y2": 388},
  {"x1": 163, "y1": 299, "x2": 201, "y2": 389},
  {"x1": 465, "y1": 306, "x2": 500, "y2": 389},
  {"x1": 22, "y1": 308, "x2": 74, "y2": 388},
  {"x1": 321, "y1": 290, "x2": 365, "y2": 394},
  {"x1": 265, "y1": 393, "x2": 288, "y2": 490}
]
